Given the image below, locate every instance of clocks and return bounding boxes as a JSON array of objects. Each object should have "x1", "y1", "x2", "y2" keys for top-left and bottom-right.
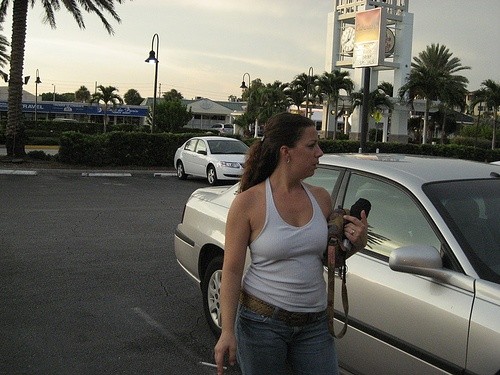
[{"x1": 341, "y1": 26, "x2": 355, "y2": 52}]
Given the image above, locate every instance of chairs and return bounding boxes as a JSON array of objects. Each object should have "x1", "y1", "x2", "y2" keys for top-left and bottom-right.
[{"x1": 445, "y1": 199, "x2": 500, "y2": 265}]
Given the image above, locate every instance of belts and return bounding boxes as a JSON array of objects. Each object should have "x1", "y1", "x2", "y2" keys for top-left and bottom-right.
[{"x1": 239, "y1": 288, "x2": 324, "y2": 323}]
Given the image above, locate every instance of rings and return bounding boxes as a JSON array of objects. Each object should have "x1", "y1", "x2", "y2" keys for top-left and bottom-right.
[{"x1": 352, "y1": 230, "x2": 355, "y2": 234}]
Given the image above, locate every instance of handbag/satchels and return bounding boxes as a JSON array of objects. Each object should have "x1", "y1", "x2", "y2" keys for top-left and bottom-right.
[{"x1": 321, "y1": 205, "x2": 351, "y2": 269}]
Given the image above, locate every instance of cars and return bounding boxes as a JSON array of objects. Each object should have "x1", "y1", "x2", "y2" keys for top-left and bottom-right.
[
  {"x1": 210, "y1": 122, "x2": 234, "y2": 135},
  {"x1": 174, "y1": 136, "x2": 250, "y2": 186},
  {"x1": 173, "y1": 153, "x2": 499, "y2": 374}
]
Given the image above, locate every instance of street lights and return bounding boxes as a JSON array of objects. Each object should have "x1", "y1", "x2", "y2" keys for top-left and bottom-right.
[
  {"x1": 305, "y1": 67, "x2": 319, "y2": 117},
  {"x1": 144, "y1": 33, "x2": 160, "y2": 133},
  {"x1": 35, "y1": 69, "x2": 42, "y2": 120},
  {"x1": 240, "y1": 73, "x2": 250, "y2": 101}
]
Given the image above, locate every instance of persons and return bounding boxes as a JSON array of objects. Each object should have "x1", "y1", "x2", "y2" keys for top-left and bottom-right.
[{"x1": 214, "y1": 113, "x2": 368, "y2": 375}]
